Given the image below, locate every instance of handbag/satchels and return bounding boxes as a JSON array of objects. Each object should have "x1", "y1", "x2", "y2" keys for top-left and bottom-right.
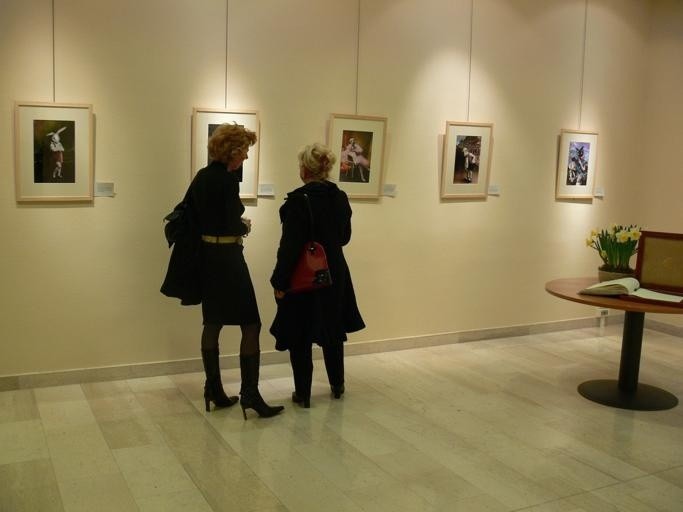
[{"x1": 285, "y1": 241, "x2": 334, "y2": 292}]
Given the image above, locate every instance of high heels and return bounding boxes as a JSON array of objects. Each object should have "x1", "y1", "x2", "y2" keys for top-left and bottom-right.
[
  {"x1": 292, "y1": 392, "x2": 310, "y2": 408},
  {"x1": 331, "y1": 385, "x2": 344, "y2": 398}
]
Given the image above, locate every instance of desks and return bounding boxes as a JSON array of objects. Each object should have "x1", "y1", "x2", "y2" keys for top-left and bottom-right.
[{"x1": 545, "y1": 277, "x2": 683, "y2": 412}]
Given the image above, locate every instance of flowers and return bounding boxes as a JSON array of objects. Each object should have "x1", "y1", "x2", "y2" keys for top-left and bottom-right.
[{"x1": 585, "y1": 223, "x2": 642, "y2": 268}]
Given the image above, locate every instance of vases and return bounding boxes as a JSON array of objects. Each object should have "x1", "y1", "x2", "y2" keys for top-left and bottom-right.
[{"x1": 597, "y1": 270, "x2": 632, "y2": 284}]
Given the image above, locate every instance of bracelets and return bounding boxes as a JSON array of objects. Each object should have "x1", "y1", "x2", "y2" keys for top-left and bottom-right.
[{"x1": 242, "y1": 220, "x2": 250, "y2": 239}]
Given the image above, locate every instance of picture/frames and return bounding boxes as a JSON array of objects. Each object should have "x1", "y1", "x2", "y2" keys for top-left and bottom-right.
[
  {"x1": 439, "y1": 119, "x2": 494, "y2": 200},
  {"x1": 328, "y1": 111, "x2": 388, "y2": 201},
  {"x1": 191, "y1": 108, "x2": 261, "y2": 201},
  {"x1": 13, "y1": 99, "x2": 96, "y2": 204},
  {"x1": 555, "y1": 129, "x2": 598, "y2": 200}
]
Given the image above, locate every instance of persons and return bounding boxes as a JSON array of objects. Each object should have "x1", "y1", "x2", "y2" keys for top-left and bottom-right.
[
  {"x1": 180, "y1": 121, "x2": 284, "y2": 419},
  {"x1": 268, "y1": 142, "x2": 367, "y2": 407},
  {"x1": 573, "y1": 151, "x2": 588, "y2": 187},
  {"x1": 50, "y1": 134, "x2": 66, "y2": 178},
  {"x1": 462, "y1": 147, "x2": 476, "y2": 182},
  {"x1": 344, "y1": 135, "x2": 370, "y2": 182}
]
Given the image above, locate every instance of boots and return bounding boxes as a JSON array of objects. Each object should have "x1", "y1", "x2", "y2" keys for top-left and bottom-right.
[
  {"x1": 240, "y1": 351, "x2": 284, "y2": 421},
  {"x1": 201, "y1": 347, "x2": 239, "y2": 412}
]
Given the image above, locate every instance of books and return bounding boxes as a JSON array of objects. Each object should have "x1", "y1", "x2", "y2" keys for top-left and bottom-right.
[{"x1": 577, "y1": 277, "x2": 682, "y2": 305}]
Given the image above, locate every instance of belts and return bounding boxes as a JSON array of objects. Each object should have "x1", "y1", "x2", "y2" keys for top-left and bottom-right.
[{"x1": 202, "y1": 235, "x2": 246, "y2": 247}]
